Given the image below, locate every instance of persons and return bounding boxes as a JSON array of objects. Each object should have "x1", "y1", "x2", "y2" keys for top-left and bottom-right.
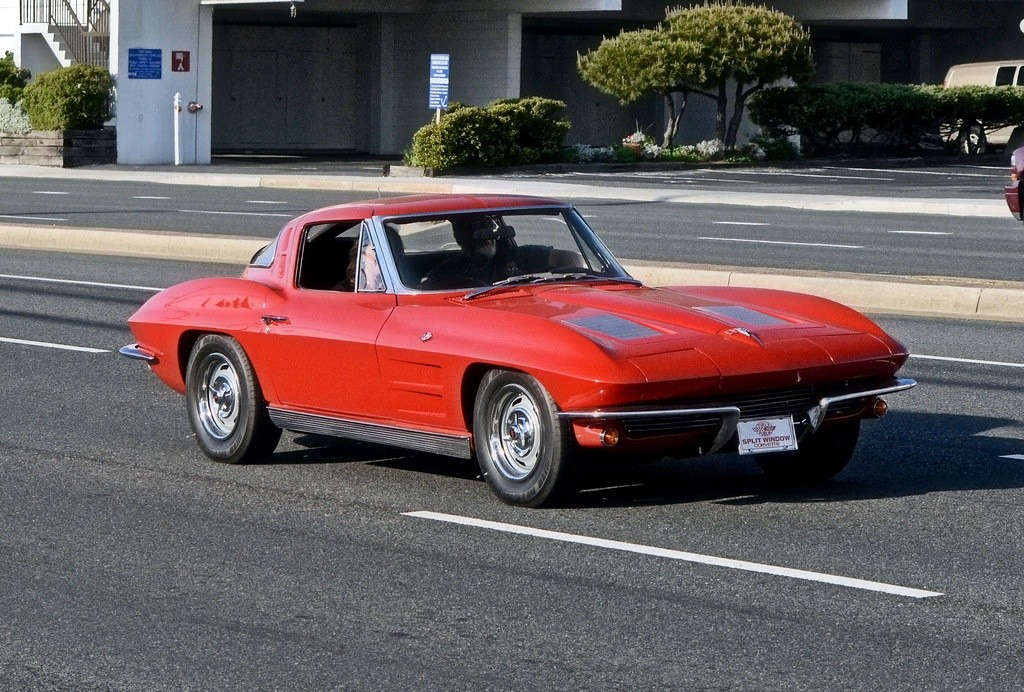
[{"x1": 349, "y1": 215, "x2": 585, "y2": 296}]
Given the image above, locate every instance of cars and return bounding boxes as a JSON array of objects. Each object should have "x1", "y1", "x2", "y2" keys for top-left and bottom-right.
[{"x1": 118, "y1": 194, "x2": 916, "y2": 509}]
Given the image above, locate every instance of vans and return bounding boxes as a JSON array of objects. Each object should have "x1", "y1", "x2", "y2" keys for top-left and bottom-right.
[{"x1": 938, "y1": 59, "x2": 1024, "y2": 155}]
